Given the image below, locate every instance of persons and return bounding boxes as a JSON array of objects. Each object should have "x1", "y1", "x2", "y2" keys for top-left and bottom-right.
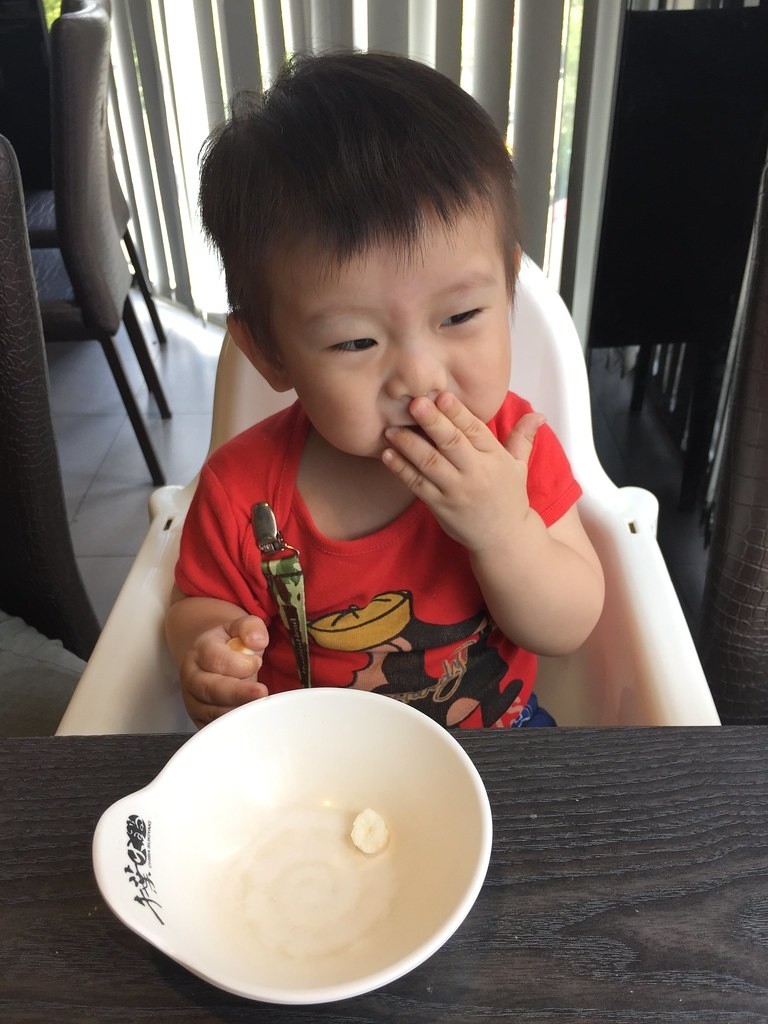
[{"x1": 163, "y1": 50, "x2": 607, "y2": 728}]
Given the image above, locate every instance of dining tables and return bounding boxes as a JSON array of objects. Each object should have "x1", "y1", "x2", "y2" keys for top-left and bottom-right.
[{"x1": 1, "y1": 723, "x2": 768, "y2": 1024}]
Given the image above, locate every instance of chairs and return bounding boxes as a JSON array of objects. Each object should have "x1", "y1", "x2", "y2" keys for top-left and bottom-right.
[
  {"x1": 51, "y1": 232, "x2": 730, "y2": 728},
  {"x1": 18, "y1": 0, "x2": 172, "y2": 483}
]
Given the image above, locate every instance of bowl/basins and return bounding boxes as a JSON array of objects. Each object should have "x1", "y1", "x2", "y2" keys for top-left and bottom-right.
[{"x1": 94, "y1": 686, "x2": 492, "y2": 1005}]
